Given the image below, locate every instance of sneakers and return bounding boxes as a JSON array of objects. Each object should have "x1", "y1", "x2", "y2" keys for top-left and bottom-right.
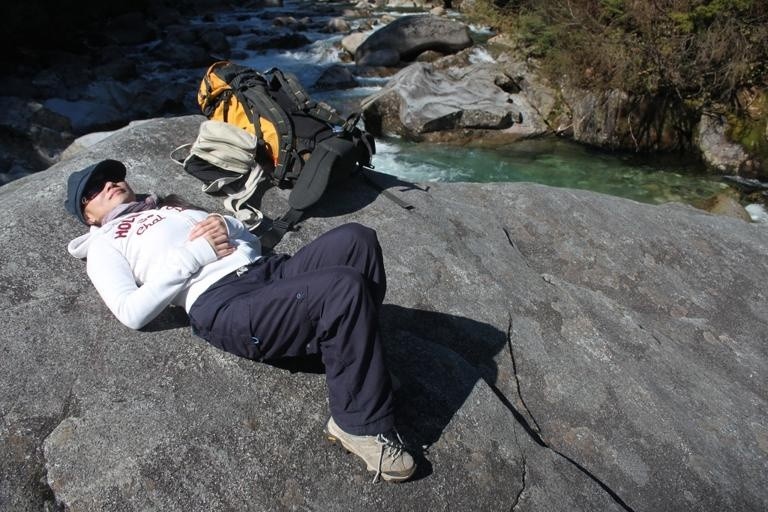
[{"x1": 322, "y1": 416, "x2": 418, "y2": 483}]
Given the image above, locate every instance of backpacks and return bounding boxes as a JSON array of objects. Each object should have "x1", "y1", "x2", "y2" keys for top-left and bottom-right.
[{"x1": 196, "y1": 60, "x2": 377, "y2": 211}]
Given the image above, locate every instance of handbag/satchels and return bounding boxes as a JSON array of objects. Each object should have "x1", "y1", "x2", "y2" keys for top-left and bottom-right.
[{"x1": 169, "y1": 120, "x2": 265, "y2": 194}]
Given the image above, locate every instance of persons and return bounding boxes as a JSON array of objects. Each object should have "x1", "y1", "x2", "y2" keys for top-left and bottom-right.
[{"x1": 64, "y1": 159, "x2": 428, "y2": 487}]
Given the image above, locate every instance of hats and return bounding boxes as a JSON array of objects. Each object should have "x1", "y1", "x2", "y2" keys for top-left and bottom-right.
[{"x1": 65, "y1": 159, "x2": 126, "y2": 225}]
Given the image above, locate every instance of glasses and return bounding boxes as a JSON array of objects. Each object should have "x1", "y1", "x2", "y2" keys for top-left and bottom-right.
[{"x1": 81, "y1": 173, "x2": 123, "y2": 209}]
